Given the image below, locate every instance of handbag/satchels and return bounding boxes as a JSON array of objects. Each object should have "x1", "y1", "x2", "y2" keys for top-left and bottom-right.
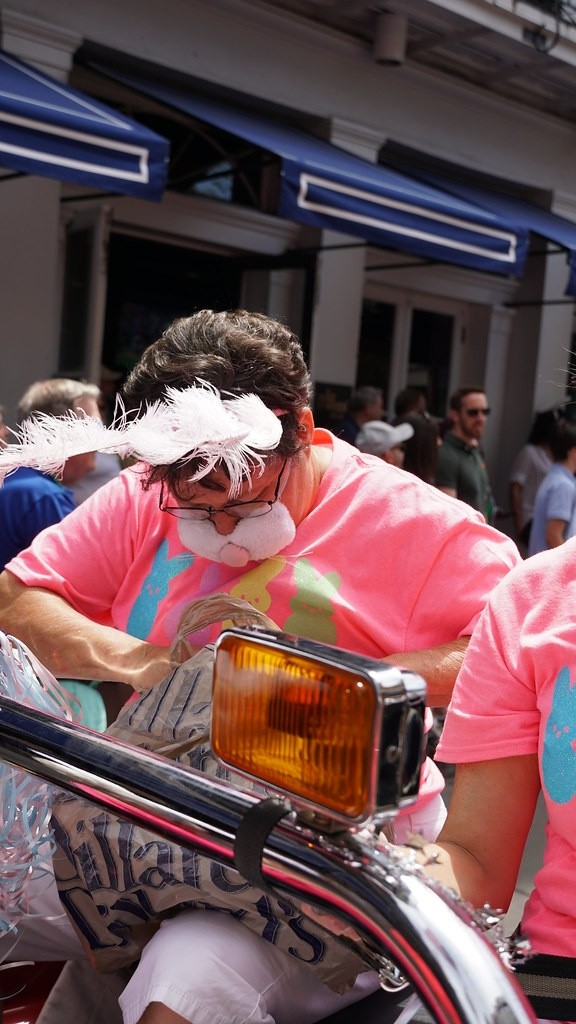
[{"x1": 48, "y1": 592, "x2": 396, "y2": 993}]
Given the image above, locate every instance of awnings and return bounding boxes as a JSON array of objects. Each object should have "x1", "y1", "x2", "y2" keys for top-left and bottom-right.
[
  {"x1": 0, "y1": 48, "x2": 169, "y2": 203},
  {"x1": 415, "y1": 166, "x2": 575, "y2": 297},
  {"x1": 96, "y1": 59, "x2": 529, "y2": 281}
]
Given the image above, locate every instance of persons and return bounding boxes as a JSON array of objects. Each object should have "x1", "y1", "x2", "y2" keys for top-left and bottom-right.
[{"x1": 0, "y1": 308, "x2": 576, "y2": 1024}]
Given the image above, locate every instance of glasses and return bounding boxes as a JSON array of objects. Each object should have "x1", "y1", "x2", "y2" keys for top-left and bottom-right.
[
  {"x1": 464, "y1": 407, "x2": 490, "y2": 417},
  {"x1": 158, "y1": 456, "x2": 288, "y2": 520}
]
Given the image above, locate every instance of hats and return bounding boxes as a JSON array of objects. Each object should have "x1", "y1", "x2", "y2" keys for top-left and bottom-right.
[{"x1": 355, "y1": 421, "x2": 415, "y2": 456}]
